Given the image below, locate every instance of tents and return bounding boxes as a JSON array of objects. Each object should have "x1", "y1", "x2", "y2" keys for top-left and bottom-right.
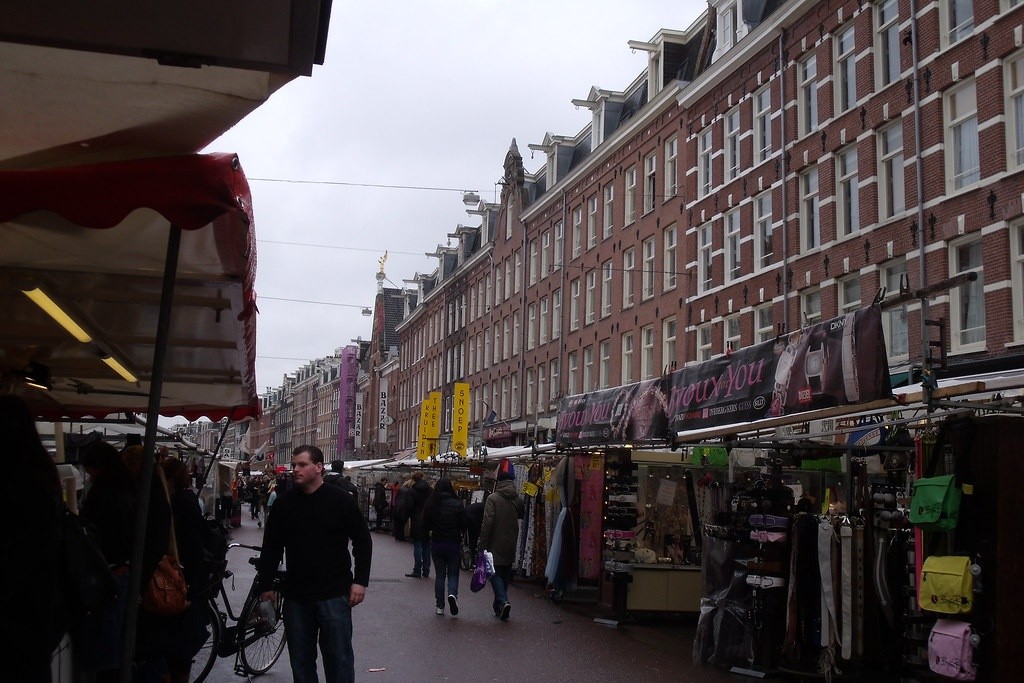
[{"x1": 0, "y1": 1, "x2": 334, "y2": 462}]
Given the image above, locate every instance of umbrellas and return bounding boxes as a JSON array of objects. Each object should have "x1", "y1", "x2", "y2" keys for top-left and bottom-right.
[{"x1": 277, "y1": 444, "x2": 552, "y2": 470}]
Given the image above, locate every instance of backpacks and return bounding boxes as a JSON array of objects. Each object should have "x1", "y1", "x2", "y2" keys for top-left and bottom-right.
[{"x1": 23, "y1": 513, "x2": 120, "y2": 620}]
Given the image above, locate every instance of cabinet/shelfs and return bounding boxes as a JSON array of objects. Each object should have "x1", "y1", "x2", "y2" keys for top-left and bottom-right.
[
  {"x1": 626, "y1": 564, "x2": 704, "y2": 611},
  {"x1": 903, "y1": 538, "x2": 933, "y2": 670}
]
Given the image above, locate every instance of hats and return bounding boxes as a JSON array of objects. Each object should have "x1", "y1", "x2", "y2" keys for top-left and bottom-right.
[{"x1": 494, "y1": 457, "x2": 516, "y2": 480}]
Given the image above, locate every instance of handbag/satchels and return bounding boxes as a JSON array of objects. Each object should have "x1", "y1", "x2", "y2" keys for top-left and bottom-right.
[
  {"x1": 919, "y1": 555, "x2": 975, "y2": 615},
  {"x1": 470, "y1": 554, "x2": 487, "y2": 593},
  {"x1": 483, "y1": 549, "x2": 496, "y2": 578},
  {"x1": 145, "y1": 554, "x2": 191, "y2": 617},
  {"x1": 928, "y1": 618, "x2": 976, "y2": 681},
  {"x1": 908, "y1": 474, "x2": 961, "y2": 530}
]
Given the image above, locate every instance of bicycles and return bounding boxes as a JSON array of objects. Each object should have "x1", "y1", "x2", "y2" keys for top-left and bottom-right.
[{"x1": 192, "y1": 543, "x2": 293, "y2": 683}]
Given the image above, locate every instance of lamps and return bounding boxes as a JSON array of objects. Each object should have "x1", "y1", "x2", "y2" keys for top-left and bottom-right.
[
  {"x1": 18, "y1": 279, "x2": 95, "y2": 343},
  {"x1": 25, "y1": 360, "x2": 53, "y2": 391},
  {"x1": 101, "y1": 353, "x2": 140, "y2": 383}
]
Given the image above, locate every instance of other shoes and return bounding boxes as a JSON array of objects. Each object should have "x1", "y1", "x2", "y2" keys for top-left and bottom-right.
[
  {"x1": 177, "y1": 672, "x2": 189, "y2": 683},
  {"x1": 258, "y1": 522, "x2": 262, "y2": 528},
  {"x1": 254, "y1": 513, "x2": 258, "y2": 519},
  {"x1": 251, "y1": 514, "x2": 254, "y2": 519},
  {"x1": 159, "y1": 672, "x2": 172, "y2": 683},
  {"x1": 405, "y1": 573, "x2": 421, "y2": 578}
]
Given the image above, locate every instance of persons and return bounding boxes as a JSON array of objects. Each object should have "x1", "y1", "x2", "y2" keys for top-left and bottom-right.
[
  {"x1": 665, "y1": 531, "x2": 682, "y2": 561},
  {"x1": 230, "y1": 460, "x2": 361, "y2": 527},
  {"x1": 257, "y1": 446, "x2": 373, "y2": 683},
  {"x1": 76, "y1": 440, "x2": 211, "y2": 683},
  {"x1": 0, "y1": 394, "x2": 64, "y2": 681},
  {"x1": 372, "y1": 455, "x2": 525, "y2": 621}
]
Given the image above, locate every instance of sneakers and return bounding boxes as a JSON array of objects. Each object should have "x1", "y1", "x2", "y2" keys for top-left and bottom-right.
[
  {"x1": 436, "y1": 608, "x2": 444, "y2": 615},
  {"x1": 448, "y1": 594, "x2": 458, "y2": 615},
  {"x1": 500, "y1": 602, "x2": 511, "y2": 621},
  {"x1": 495, "y1": 612, "x2": 501, "y2": 616}
]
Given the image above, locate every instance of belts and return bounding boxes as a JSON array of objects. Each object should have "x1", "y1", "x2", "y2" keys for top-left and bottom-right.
[
  {"x1": 605, "y1": 540, "x2": 634, "y2": 550},
  {"x1": 608, "y1": 486, "x2": 637, "y2": 492},
  {"x1": 608, "y1": 494, "x2": 637, "y2": 503},
  {"x1": 818, "y1": 514, "x2": 845, "y2": 648},
  {"x1": 606, "y1": 475, "x2": 638, "y2": 484},
  {"x1": 607, "y1": 505, "x2": 638, "y2": 517},
  {"x1": 603, "y1": 530, "x2": 636, "y2": 539},
  {"x1": 604, "y1": 561, "x2": 633, "y2": 572},
  {"x1": 854, "y1": 518, "x2": 865, "y2": 657},
  {"x1": 604, "y1": 551, "x2": 635, "y2": 560},
  {"x1": 841, "y1": 515, "x2": 851, "y2": 660}
]
